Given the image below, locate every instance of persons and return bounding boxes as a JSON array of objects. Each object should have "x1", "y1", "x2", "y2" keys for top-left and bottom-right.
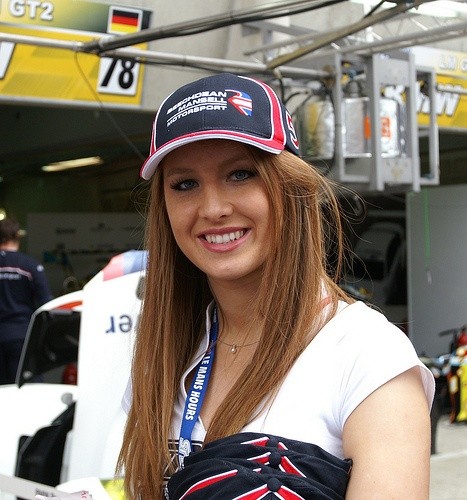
[
  {"x1": 0, "y1": 219, "x2": 52, "y2": 386},
  {"x1": 122, "y1": 73, "x2": 436, "y2": 500}
]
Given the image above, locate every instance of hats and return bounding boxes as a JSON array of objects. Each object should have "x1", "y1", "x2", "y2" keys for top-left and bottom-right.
[
  {"x1": 167, "y1": 432, "x2": 352, "y2": 500},
  {"x1": 139, "y1": 72, "x2": 299, "y2": 180}
]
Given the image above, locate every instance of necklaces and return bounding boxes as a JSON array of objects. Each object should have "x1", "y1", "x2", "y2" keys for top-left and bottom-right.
[{"x1": 218, "y1": 338, "x2": 262, "y2": 354}]
